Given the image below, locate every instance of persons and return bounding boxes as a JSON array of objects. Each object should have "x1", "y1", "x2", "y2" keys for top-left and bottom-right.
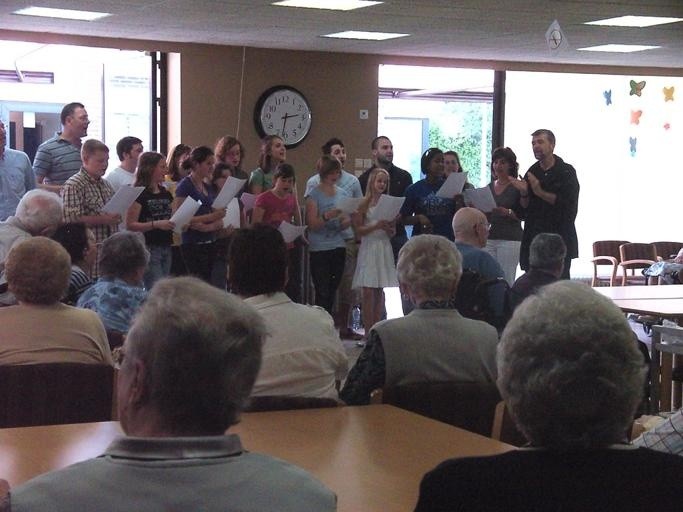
[
  {"x1": 339, "y1": 234, "x2": 499, "y2": 407},
  {"x1": 1, "y1": 275, "x2": 338, "y2": 511},
  {"x1": 225, "y1": 225, "x2": 351, "y2": 401},
  {"x1": 414, "y1": 282, "x2": 683, "y2": 511},
  {"x1": 631, "y1": 406, "x2": 683, "y2": 456},
  {"x1": 644, "y1": 247, "x2": 683, "y2": 285},
  {"x1": 1, "y1": 103, "x2": 579, "y2": 362}
]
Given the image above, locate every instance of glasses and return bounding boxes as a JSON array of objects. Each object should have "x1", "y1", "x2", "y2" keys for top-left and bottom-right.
[
  {"x1": 473, "y1": 222, "x2": 492, "y2": 231},
  {"x1": 110, "y1": 346, "x2": 128, "y2": 370},
  {"x1": 88, "y1": 241, "x2": 104, "y2": 250}
]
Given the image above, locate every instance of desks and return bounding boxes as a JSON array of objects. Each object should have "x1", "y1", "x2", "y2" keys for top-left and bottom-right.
[
  {"x1": 0, "y1": 404, "x2": 523, "y2": 510},
  {"x1": 591, "y1": 284, "x2": 683, "y2": 412}
]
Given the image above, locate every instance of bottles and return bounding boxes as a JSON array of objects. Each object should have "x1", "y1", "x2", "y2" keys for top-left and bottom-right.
[{"x1": 352, "y1": 305, "x2": 360, "y2": 331}]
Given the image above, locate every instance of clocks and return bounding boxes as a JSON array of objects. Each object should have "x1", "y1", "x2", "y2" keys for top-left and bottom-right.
[{"x1": 253, "y1": 85, "x2": 312, "y2": 149}]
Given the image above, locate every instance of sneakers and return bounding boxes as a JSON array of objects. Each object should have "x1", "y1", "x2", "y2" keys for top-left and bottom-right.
[
  {"x1": 638, "y1": 315, "x2": 658, "y2": 323},
  {"x1": 340, "y1": 328, "x2": 363, "y2": 340},
  {"x1": 629, "y1": 313, "x2": 642, "y2": 322}
]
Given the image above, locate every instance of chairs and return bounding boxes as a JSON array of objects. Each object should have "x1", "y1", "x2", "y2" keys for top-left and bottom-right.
[
  {"x1": 647, "y1": 241, "x2": 683, "y2": 285},
  {"x1": 241, "y1": 395, "x2": 340, "y2": 412},
  {"x1": 618, "y1": 242, "x2": 662, "y2": 285},
  {"x1": 368, "y1": 379, "x2": 507, "y2": 441},
  {"x1": 649, "y1": 324, "x2": 683, "y2": 415},
  {"x1": 0, "y1": 361, "x2": 114, "y2": 427},
  {"x1": 590, "y1": 239, "x2": 631, "y2": 286}
]
